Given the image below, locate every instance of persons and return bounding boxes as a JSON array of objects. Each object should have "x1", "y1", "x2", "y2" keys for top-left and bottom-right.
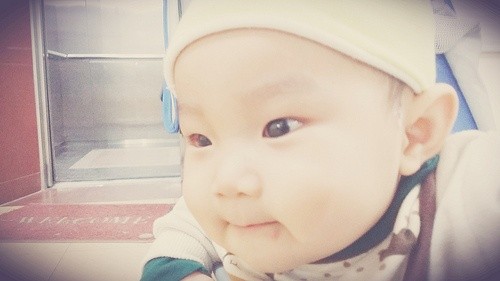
[{"x1": 140, "y1": 1, "x2": 499, "y2": 281}]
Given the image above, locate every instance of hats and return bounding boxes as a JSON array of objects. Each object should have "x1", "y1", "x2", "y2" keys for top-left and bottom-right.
[{"x1": 162, "y1": 1, "x2": 434, "y2": 117}]
